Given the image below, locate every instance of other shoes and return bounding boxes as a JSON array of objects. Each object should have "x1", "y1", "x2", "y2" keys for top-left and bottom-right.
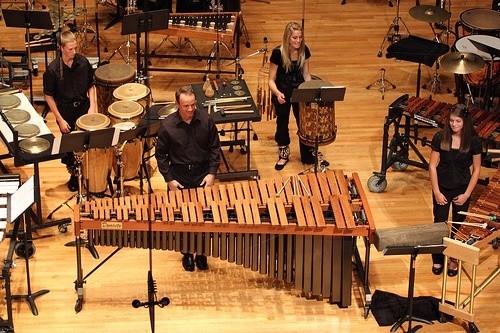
[
  {"x1": 433, "y1": 264, "x2": 444, "y2": 275},
  {"x1": 275, "y1": 158, "x2": 289, "y2": 170},
  {"x1": 448, "y1": 265, "x2": 458, "y2": 277},
  {"x1": 311, "y1": 157, "x2": 329, "y2": 166}
]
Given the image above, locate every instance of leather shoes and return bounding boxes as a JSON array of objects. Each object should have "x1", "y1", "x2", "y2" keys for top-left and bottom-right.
[
  {"x1": 196, "y1": 255, "x2": 208, "y2": 270},
  {"x1": 183, "y1": 253, "x2": 195, "y2": 271},
  {"x1": 68, "y1": 175, "x2": 78, "y2": 192}
]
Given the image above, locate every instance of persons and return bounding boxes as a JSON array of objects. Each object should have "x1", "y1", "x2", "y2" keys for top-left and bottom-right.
[
  {"x1": 43, "y1": 30, "x2": 97, "y2": 193},
  {"x1": 153, "y1": 87, "x2": 221, "y2": 272},
  {"x1": 429, "y1": 103, "x2": 482, "y2": 277},
  {"x1": 266, "y1": 22, "x2": 330, "y2": 172}
]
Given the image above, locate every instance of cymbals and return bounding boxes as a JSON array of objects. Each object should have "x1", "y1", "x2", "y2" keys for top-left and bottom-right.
[
  {"x1": 408, "y1": 5, "x2": 451, "y2": 23},
  {"x1": 158, "y1": 103, "x2": 179, "y2": 117},
  {"x1": 440, "y1": 51, "x2": 485, "y2": 74},
  {"x1": 13, "y1": 123, "x2": 40, "y2": 138},
  {"x1": 19, "y1": 137, "x2": 50, "y2": 154},
  {"x1": 0, "y1": 95, "x2": 21, "y2": 109},
  {"x1": 4, "y1": 108, "x2": 31, "y2": 124}
]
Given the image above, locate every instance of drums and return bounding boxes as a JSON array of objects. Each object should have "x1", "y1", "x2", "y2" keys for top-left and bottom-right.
[
  {"x1": 112, "y1": 122, "x2": 142, "y2": 180},
  {"x1": 297, "y1": 79, "x2": 338, "y2": 147},
  {"x1": 94, "y1": 62, "x2": 136, "y2": 118},
  {"x1": 454, "y1": 34, "x2": 500, "y2": 87},
  {"x1": 112, "y1": 83, "x2": 152, "y2": 117},
  {"x1": 458, "y1": 8, "x2": 500, "y2": 38},
  {"x1": 107, "y1": 100, "x2": 144, "y2": 127},
  {"x1": 76, "y1": 113, "x2": 113, "y2": 194}
]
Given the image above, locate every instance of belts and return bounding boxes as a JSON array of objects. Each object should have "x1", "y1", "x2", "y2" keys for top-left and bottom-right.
[
  {"x1": 61, "y1": 95, "x2": 87, "y2": 107},
  {"x1": 179, "y1": 164, "x2": 201, "y2": 171}
]
